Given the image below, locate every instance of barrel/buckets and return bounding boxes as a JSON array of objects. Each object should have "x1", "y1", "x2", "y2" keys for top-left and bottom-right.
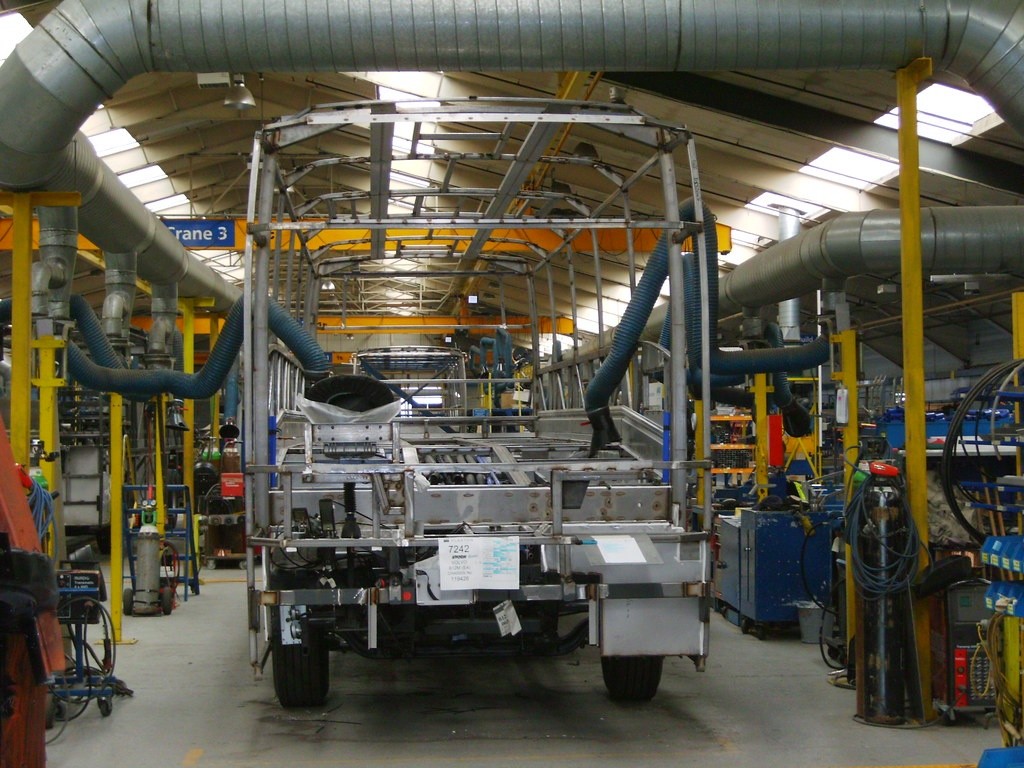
[{"x1": 797, "y1": 601, "x2": 833, "y2": 643}]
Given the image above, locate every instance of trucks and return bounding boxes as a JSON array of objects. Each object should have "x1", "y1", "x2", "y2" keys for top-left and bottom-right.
[{"x1": 231, "y1": 101, "x2": 717, "y2": 709}]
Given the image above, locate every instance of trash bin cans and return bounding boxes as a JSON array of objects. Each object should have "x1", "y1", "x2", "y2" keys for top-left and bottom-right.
[{"x1": 795, "y1": 600, "x2": 834, "y2": 644}]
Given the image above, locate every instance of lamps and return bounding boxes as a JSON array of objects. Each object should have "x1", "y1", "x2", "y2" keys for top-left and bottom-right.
[
  {"x1": 273, "y1": 169, "x2": 294, "y2": 192},
  {"x1": 321, "y1": 273, "x2": 335, "y2": 290},
  {"x1": 572, "y1": 142, "x2": 598, "y2": 159},
  {"x1": 224, "y1": 72, "x2": 256, "y2": 110},
  {"x1": 608, "y1": 85, "x2": 632, "y2": 114}
]
